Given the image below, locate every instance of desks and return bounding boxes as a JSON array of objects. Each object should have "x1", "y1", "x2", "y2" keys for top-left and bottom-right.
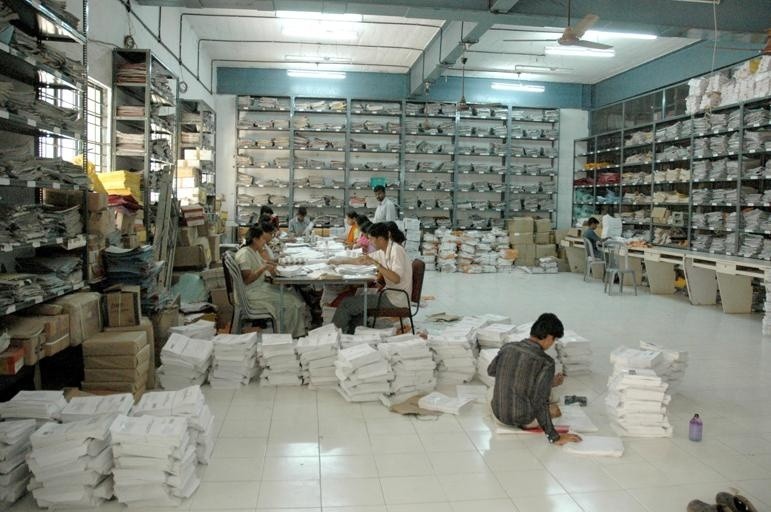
[{"x1": 274, "y1": 231, "x2": 372, "y2": 332}]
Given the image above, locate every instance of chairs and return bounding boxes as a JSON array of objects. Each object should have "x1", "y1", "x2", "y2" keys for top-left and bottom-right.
[
  {"x1": 225, "y1": 252, "x2": 279, "y2": 336},
  {"x1": 368, "y1": 259, "x2": 426, "y2": 335},
  {"x1": 602, "y1": 242, "x2": 637, "y2": 297}
]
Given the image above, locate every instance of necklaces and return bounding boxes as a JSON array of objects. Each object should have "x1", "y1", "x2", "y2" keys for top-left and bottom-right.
[{"x1": 260, "y1": 248, "x2": 265, "y2": 259}]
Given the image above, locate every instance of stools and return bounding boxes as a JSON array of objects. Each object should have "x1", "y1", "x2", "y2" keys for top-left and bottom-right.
[
  {"x1": 717, "y1": 273, "x2": 752, "y2": 314},
  {"x1": 589, "y1": 250, "x2": 603, "y2": 280},
  {"x1": 644, "y1": 260, "x2": 675, "y2": 295},
  {"x1": 683, "y1": 267, "x2": 717, "y2": 304},
  {"x1": 615, "y1": 256, "x2": 642, "y2": 286},
  {"x1": 564, "y1": 246, "x2": 589, "y2": 273}
]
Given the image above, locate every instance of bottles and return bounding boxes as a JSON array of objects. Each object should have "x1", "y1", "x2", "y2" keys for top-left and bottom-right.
[{"x1": 689, "y1": 414, "x2": 702, "y2": 443}]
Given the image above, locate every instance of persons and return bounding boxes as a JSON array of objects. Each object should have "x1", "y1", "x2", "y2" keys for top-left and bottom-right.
[
  {"x1": 583, "y1": 216, "x2": 613, "y2": 263},
  {"x1": 352, "y1": 215, "x2": 372, "y2": 241},
  {"x1": 371, "y1": 185, "x2": 395, "y2": 224},
  {"x1": 259, "y1": 206, "x2": 283, "y2": 262},
  {"x1": 488, "y1": 312, "x2": 583, "y2": 445},
  {"x1": 335, "y1": 211, "x2": 360, "y2": 245},
  {"x1": 352, "y1": 221, "x2": 376, "y2": 252},
  {"x1": 234, "y1": 223, "x2": 311, "y2": 338},
  {"x1": 327, "y1": 222, "x2": 413, "y2": 335},
  {"x1": 288, "y1": 207, "x2": 313, "y2": 237}
]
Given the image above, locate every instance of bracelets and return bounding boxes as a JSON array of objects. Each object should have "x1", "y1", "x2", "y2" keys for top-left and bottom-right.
[{"x1": 380, "y1": 269, "x2": 387, "y2": 274}]
